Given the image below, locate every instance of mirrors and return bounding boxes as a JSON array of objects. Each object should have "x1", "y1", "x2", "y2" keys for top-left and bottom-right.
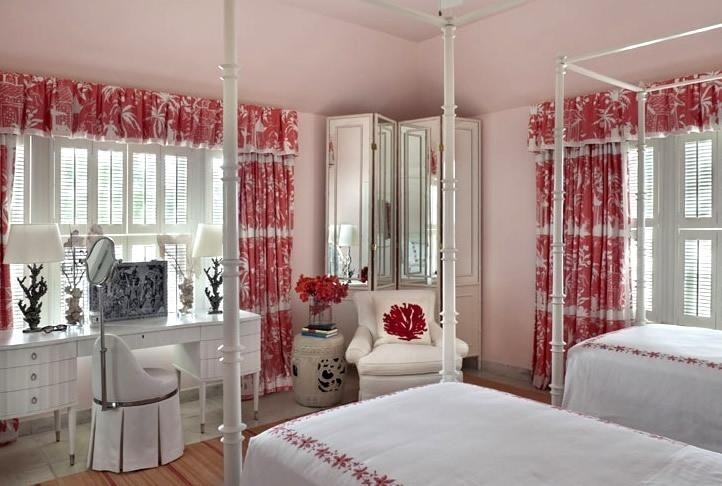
[
  {"x1": 78, "y1": 236, "x2": 125, "y2": 412},
  {"x1": 324, "y1": 113, "x2": 482, "y2": 290}
]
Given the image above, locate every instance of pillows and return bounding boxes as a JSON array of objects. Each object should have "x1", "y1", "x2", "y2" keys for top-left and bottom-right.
[{"x1": 366, "y1": 289, "x2": 434, "y2": 348}]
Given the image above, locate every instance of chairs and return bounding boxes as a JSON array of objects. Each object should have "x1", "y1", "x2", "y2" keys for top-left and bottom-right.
[
  {"x1": 83, "y1": 333, "x2": 187, "y2": 476},
  {"x1": 336, "y1": 288, "x2": 471, "y2": 405}
]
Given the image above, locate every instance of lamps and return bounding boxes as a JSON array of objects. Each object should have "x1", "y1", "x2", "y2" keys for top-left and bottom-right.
[
  {"x1": 190, "y1": 222, "x2": 226, "y2": 317},
  {"x1": 2, "y1": 222, "x2": 68, "y2": 334}
]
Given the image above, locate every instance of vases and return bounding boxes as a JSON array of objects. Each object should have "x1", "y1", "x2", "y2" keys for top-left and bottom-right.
[{"x1": 306, "y1": 294, "x2": 334, "y2": 324}]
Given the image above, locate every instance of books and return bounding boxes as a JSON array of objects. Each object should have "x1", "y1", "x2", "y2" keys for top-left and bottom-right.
[{"x1": 301, "y1": 323, "x2": 339, "y2": 338}]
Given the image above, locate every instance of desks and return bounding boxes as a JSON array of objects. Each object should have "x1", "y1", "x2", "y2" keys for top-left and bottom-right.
[{"x1": 0, "y1": 308, "x2": 263, "y2": 468}]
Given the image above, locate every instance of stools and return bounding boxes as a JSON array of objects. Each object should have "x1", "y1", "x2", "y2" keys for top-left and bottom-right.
[{"x1": 287, "y1": 329, "x2": 347, "y2": 410}]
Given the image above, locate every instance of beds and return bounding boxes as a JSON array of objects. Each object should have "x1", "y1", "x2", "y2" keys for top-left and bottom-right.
[
  {"x1": 550, "y1": 18, "x2": 721, "y2": 455},
  {"x1": 216, "y1": 0, "x2": 721, "y2": 486}
]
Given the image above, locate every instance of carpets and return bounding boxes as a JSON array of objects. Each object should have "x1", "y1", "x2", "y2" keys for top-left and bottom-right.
[{"x1": 0, "y1": 373, "x2": 360, "y2": 485}]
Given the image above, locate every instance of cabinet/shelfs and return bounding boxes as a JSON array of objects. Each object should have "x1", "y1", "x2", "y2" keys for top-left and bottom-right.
[{"x1": 323, "y1": 282, "x2": 484, "y2": 370}]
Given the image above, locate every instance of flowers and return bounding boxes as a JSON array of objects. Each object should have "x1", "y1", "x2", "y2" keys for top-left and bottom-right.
[{"x1": 290, "y1": 272, "x2": 353, "y2": 317}]
